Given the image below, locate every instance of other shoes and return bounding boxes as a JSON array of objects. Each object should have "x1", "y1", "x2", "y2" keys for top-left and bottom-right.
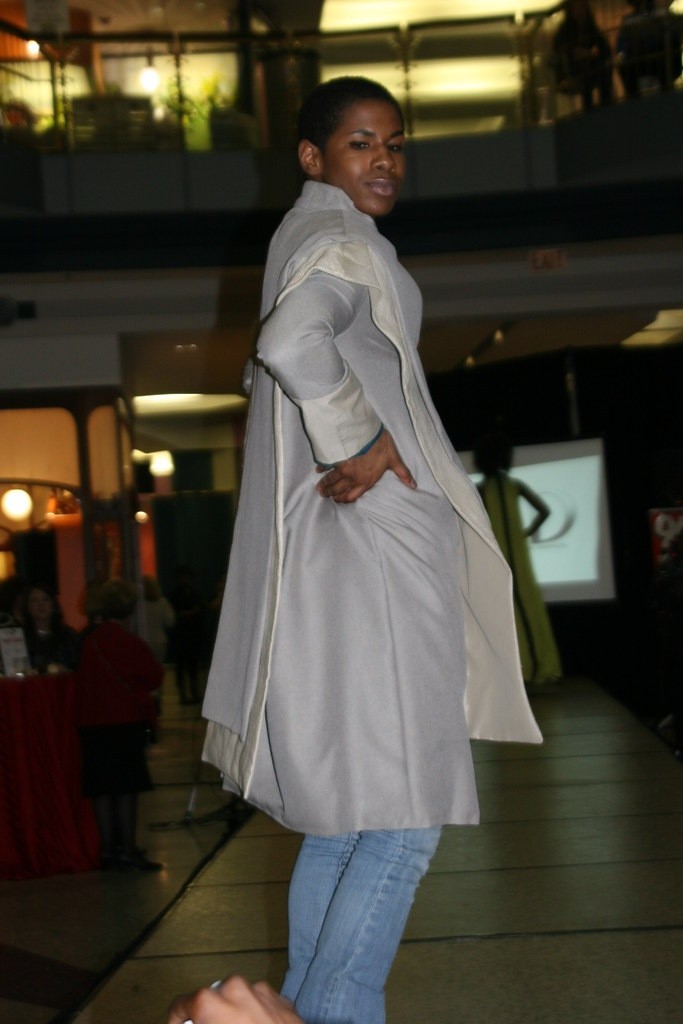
[
  {"x1": 107, "y1": 847, "x2": 163, "y2": 870},
  {"x1": 180, "y1": 695, "x2": 202, "y2": 705}
]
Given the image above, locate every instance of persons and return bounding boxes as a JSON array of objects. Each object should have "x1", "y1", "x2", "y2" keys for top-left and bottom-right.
[
  {"x1": 616, "y1": 1, "x2": 683, "y2": 99},
  {"x1": 5, "y1": 584, "x2": 74, "y2": 676},
  {"x1": 545, "y1": 1, "x2": 615, "y2": 108},
  {"x1": 472, "y1": 435, "x2": 563, "y2": 682},
  {"x1": 76, "y1": 585, "x2": 160, "y2": 876},
  {"x1": 203, "y1": 78, "x2": 545, "y2": 1024},
  {"x1": 232, "y1": 20, "x2": 316, "y2": 141}
]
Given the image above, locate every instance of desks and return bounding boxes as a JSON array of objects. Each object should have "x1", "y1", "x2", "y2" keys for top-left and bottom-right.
[{"x1": 0, "y1": 673, "x2": 106, "y2": 878}]
